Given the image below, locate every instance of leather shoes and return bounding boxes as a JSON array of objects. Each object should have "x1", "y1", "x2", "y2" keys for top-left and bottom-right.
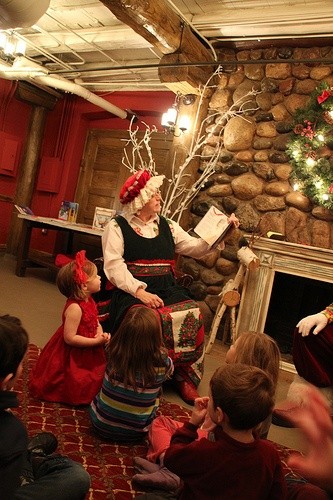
[{"x1": 179, "y1": 381, "x2": 200, "y2": 401}]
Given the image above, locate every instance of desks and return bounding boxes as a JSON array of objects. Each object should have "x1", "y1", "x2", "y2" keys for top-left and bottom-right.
[{"x1": 14, "y1": 213, "x2": 102, "y2": 277}]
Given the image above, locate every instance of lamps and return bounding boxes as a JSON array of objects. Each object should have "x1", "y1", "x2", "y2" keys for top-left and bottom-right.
[
  {"x1": 161, "y1": 108, "x2": 189, "y2": 137},
  {"x1": 0, "y1": 32, "x2": 26, "y2": 64}
]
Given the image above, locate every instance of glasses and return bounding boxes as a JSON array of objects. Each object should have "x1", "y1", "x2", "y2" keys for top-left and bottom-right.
[{"x1": 150, "y1": 190, "x2": 162, "y2": 200}]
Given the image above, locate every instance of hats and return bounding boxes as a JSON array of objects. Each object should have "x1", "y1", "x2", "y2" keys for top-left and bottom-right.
[{"x1": 119, "y1": 169, "x2": 165, "y2": 217}]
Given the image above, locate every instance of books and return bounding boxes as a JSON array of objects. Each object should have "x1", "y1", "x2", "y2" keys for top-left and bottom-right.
[
  {"x1": 16, "y1": 204, "x2": 34, "y2": 216},
  {"x1": 194, "y1": 205, "x2": 233, "y2": 251},
  {"x1": 58, "y1": 201, "x2": 79, "y2": 223}
]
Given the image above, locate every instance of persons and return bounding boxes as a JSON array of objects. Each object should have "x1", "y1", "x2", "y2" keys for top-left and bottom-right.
[
  {"x1": 87, "y1": 304, "x2": 174, "y2": 444},
  {"x1": 277, "y1": 385, "x2": 332, "y2": 490},
  {"x1": 101, "y1": 169, "x2": 240, "y2": 405},
  {"x1": 131, "y1": 331, "x2": 280, "y2": 490},
  {"x1": 270, "y1": 303, "x2": 332, "y2": 428},
  {"x1": 0, "y1": 314, "x2": 91, "y2": 500},
  {"x1": 31, "y1": 250, "x2": 108, "y2": 404},
  {"x1": 161, "y1": 363, "x2": 282, "y2": 500}
]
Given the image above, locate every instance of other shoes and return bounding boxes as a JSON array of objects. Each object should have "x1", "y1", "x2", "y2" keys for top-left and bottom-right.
[{"x1": 27, "y1": 432, "x2": 58, "y2": 456}]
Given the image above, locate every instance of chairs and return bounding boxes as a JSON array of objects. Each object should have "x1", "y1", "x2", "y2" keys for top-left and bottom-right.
[{"x1": 94, "y1": 257, "x2": 193, "y2": 333}]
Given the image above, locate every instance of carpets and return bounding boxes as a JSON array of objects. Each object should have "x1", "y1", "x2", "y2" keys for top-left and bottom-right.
[{"x1": 9, "y1": 343, "x2": 333, "y2": 500}]
